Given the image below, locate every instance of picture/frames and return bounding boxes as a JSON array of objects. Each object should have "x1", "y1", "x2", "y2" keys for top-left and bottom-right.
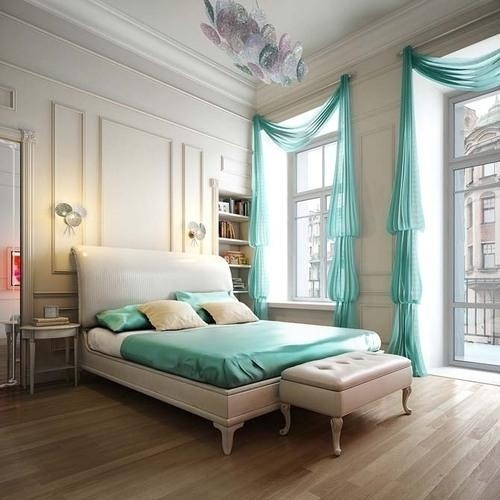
[
  {"x1": 43, "y1": 306, "x2": 58, "y2": 319},
  {"x1": 7, "y1": 246, "x2": 21, "y2": 290}
]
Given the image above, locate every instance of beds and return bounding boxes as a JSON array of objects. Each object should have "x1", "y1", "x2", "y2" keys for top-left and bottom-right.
[{"x1": 70, "y1": 245, "x2": 383, "y2": 456}]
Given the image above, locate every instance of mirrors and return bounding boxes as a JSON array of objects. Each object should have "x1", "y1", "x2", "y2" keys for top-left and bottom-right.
[{"x1": 0, "y1": 127, "x2": 34, "y2": 388}]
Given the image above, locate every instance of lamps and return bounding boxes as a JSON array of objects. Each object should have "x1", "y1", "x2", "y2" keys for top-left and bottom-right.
[
  {"x1": 200, "y1": 0, "x2": 309, "y2": 88},
  {"x1": 185, "y1": 221, "x2": 207, "y2": 249},
  {"x1": 55, "y1": 202, "x2": 87, "y2": 238}
]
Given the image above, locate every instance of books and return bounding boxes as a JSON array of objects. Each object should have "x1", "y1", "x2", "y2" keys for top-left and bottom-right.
[
  {"x1": 219, "y1": 195, "x2": 250, "y2": 291},
  {"x1": 31, "y1": 321, "x2": 69, "y2": 326},
  {"x1": 31, "y1": 317, "x2": 69, "y2": 323}
]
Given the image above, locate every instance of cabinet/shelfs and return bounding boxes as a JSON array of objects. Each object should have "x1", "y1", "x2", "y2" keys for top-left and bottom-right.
[
  {"x1": 19, "y1": 322, "x2": 80, "y2": 396},
  {"x1": 212, "y1": 186, "x2": 255, "y2": 313},
  {"x1": 1, "y1": 320, "x2": 21, "y2": 389}
]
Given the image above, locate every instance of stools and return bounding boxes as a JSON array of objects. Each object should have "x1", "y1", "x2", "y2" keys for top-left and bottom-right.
[{"x1": 278, "y1": 350, "x2": 414, "y2": 457}]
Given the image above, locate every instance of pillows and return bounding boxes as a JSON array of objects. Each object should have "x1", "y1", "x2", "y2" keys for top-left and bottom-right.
[{"x1": 95, "y1": 289, "x2": 260, "y2": 332}]
